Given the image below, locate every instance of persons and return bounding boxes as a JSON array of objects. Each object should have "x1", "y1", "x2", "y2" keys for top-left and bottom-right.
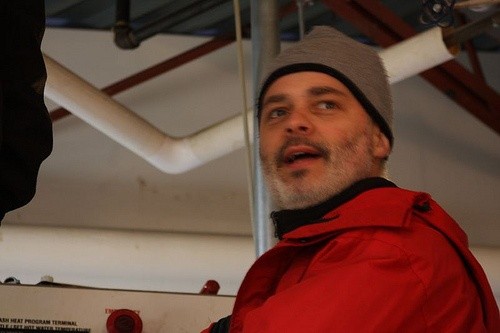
[{"x1": 196, "y1": 24, "x2": 500, "y2": 332}]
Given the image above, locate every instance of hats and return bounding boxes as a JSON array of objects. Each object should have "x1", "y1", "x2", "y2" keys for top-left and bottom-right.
[{"x1": 257, "y1": 25, "x2": 394, "y2": 145}]
